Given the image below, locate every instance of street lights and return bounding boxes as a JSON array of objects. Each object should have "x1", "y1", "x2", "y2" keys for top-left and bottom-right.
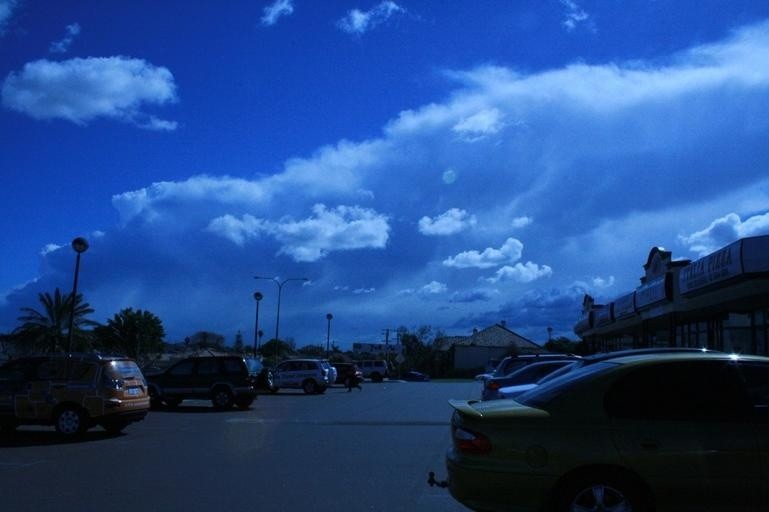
[
  {"x1": 253, "y1": 276, "x2": 309, "y2": 340},
  {"x1": 253, "y1": 292, "x2": 263, "y2": 360},
  {"x1": 66, "y1": 238, "x2": 89, "y2": 356},
  {"x1": 325, "y1": 313, "x2": 333, "y2": 354}
]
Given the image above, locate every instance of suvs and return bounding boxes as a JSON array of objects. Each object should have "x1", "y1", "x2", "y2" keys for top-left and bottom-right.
[
  {"x1": 0, "y1": 349, "x2": 151, "y2": 443},
  {"x1": 273, "y1": 357, "x2": 390, "y2": 394},
  {"x1": 142, "y1": 353, "x2": 275, "y2": 412}
]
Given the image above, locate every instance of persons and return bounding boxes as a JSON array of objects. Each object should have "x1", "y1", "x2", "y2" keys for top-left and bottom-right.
[{"x1": 345, "y1": 359, "x2": 363, "y2": 393}]
